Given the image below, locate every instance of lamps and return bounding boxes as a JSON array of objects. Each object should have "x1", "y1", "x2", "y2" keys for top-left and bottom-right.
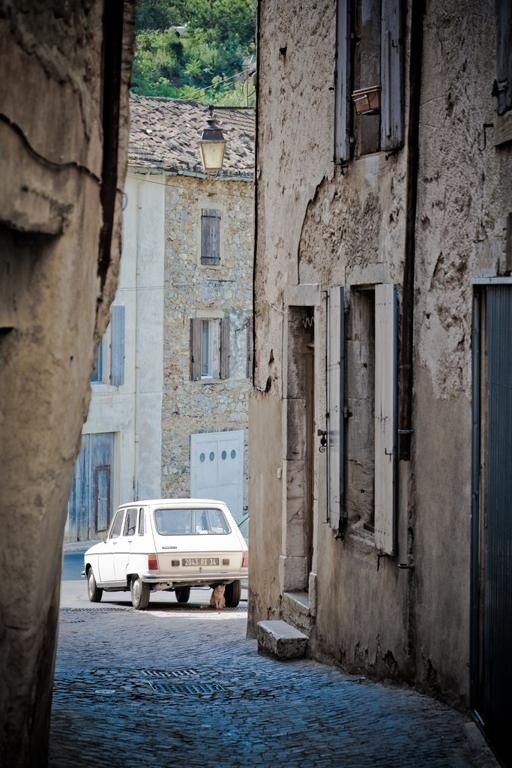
[{"x1": 196, "y1": 105, "x2": 257, "y2": 173}]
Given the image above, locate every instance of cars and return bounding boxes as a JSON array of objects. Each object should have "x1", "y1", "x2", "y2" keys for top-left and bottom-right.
[{"x1": 83, "y1": 497, "x2": 247, "y2": 611}]
